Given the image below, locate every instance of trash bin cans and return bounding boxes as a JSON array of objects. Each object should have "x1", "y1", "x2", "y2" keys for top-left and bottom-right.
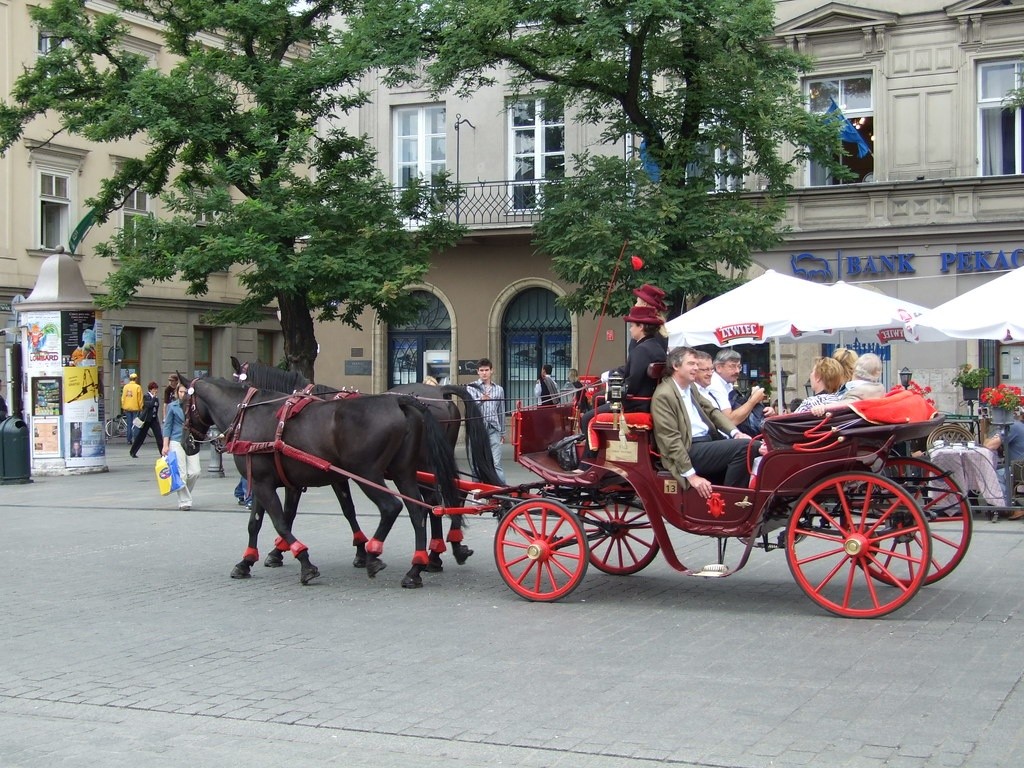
[{"x1": 0, "y1": 415, "x2": 33, "y2": 484}]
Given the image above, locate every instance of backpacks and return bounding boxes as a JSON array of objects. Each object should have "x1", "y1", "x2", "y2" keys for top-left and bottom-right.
[{"x1": 728, "y1": 386, "x2": 766, "y2": 438}]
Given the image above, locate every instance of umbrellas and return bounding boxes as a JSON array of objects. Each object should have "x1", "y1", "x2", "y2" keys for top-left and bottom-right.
[
  {"x1": 903, "y1": 263, "x2": 1024, "y2": 344},
  {"x1": 664, "y1": 269, "x2": 932, "y2": 416}
]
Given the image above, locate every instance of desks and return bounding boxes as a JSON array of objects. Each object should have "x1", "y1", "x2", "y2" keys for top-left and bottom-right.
[{"x1": 928, "y1": 440, "x2": 1006, "y2": 514}]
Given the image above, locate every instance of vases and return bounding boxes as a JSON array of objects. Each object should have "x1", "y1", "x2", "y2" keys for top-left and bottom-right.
[
  {"x1": 991, "y1": 406, "x2": 1014, "y2": 424},
  {"x1": 963, "y1": 386, "x2": 978, "y2": 400}
]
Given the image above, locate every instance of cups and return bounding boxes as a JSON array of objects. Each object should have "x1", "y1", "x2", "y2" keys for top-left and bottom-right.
[
  {"x1": 967, "y1": 440, "x2": 975, "y2": 449},
  {"x1": 935, "y1": 440, "x2": 944, "y2": 449}
]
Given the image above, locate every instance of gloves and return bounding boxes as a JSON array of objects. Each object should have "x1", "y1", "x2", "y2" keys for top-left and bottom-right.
[{"x1": 600, "y1": 370, "x2": 609, "y2": 383}]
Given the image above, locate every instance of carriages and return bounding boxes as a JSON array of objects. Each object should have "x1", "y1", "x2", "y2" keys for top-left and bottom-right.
[{"x1": 174, "y1": 355, "x2": 973, "y2": 619}]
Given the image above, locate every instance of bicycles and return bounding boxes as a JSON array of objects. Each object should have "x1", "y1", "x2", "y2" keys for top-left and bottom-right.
[{"x1": 104, "y1": 412, "x2": 155, "y2": 437}]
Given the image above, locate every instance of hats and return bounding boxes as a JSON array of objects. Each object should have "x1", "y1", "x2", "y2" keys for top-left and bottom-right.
[
  {"x1": 130, "y1": 373, "x2": 139, "y2": 378},
  {"x1": 633, "y1": 284, "x2": 667, "y2": 310},
  {"x1": 623, "y1": 306, "x2": 664, "y2": 326},
  {"x1": 168, "y1": 374, "x2": 178, "y2": 381}
]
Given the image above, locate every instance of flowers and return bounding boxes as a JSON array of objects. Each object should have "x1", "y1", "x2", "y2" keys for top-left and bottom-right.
[
  {"x1": 885, "y1": 380, "x2": 936, "y2": 408},
  {"x1": 950, "y1": 364, "x2": 991, "y2": 388},
  {"x1": 980, "y1": 383, "x2": 1024, "y2": 411}
]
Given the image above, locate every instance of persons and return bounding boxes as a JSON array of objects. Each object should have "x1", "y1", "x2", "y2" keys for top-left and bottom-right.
[
  {"x1": 568, "y1": 369, "x2": 587, "y2": 418},
  {"x1": 563, "y1": 307, "x2": 665, "y2": 474},
  {"x1": 592, "y1": 285, "x2": 669, "y2": 408},
  {"x1": 650, "y1": 347, "x2": 764, "y2": 498},
  {"x1": 534, "y1": 365, "x2": 561, "y2": 409},
  {"x1": 121, "y1": 373, "x2": 146, "y2": 445},
  {"x1": 811, "y1": 353, "x2": 885, "y2": 417},
  {"x1": 466, "y1": 358, "x2": 506, "y2": 505},
  {"x1": 772, "y1": 399, "x2": 788, "y2": 415},
  {"x1": 161, "y1": 382, "x2": 201, "y2": 511},
  {"x1": 234, "y1": 476, "x2": 252, "y2": 510},
  {"x1": 130, "y1": 380, "x2": 163, "y2": 458},
  {"x1": 162, "y1": 374, "x2": 179, "y2": 423},
  {"x1": 709, "y1": 349, "x2": 776, "y2": 426},
  {"x1": 789, "y1": 397, "x2": 804, "y2": 413},
  {"x1": 749, "y1": 357, "x2": 845, "y2": 489},
  {"x1": 694, "y1": 352, "x2": 729, "y2": 439},
  {"x1": 831, "y1": 348, "x2": 859, "y2": 396},
  {"x1": 0, "y1": 379, "x2": 8, "y2": 423},
  {"x1": 984, "y1": 420, "x2": 1024, "y2": 520},
  {"x1": 423, "y1": 375, "x2": 438, "y2": 385}
]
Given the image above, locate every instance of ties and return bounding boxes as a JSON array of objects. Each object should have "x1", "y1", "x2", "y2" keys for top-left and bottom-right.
[{"x1": 708, "y1": 392, "x2": 721, "y2": 413}]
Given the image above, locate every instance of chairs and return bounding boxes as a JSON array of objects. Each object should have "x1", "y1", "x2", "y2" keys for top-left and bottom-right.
[
  {"x1": 927, "y1": 425, "x2": 1024, "y2": 519},
  {"x1": 591, "y1": 362, "x2": 667, "y2": 432}
]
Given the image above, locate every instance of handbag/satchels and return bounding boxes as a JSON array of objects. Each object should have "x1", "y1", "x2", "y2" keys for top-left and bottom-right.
[
  {"x1": 546, "y1": 434, "x2": 586, "y2": 471},
  {"x1": 155, "y1": 450, "x2": 186, "y2": 496},
  {"x1": 133, "y1": 417, "x2": 145, "y2": 429}
]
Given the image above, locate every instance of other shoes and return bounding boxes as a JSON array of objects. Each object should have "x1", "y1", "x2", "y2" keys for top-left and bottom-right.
[
  {"x1": 179, "y1": 505, "x2": 190, "y2": 511},
  {"x1": 238, "y1": 496, "x2": 245, "y2": 505},
  {"x1": 130, "y1": 452, "x2": 138, "y2": 458},
  {"x1": 572, "y1": 468, "x2": 583, "y2": 474},
  {"x1": 244, "y1": 496, "x2": 252, "y2": 509},
  {"x1": 1009, "y1": 510, "x2": 1024, "y2": 519},
  {"x1": 128, "y1": 441, "x2": 132, "y2": 445}
]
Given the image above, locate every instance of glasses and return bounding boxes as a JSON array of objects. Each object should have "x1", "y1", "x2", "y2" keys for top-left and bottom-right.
[
  {"x1": 722, "y1": 363, "x2": 742, "y2": 372},
  {"x1": 696, "y1": 368, "x2": 715, "y2": 373}
]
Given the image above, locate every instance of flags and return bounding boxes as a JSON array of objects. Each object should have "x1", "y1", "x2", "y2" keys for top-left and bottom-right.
[
  {"x1": 69, "y1": 207, "x2": 96, "y2": 255},
  {"x1": 822, "y1": 102, "x2": 868, "y2": 158}
]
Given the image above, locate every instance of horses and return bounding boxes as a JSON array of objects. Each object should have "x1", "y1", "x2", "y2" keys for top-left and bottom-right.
[
  {"x1": 230, "y1": 356, "x2": 519, "y2": 573},
  {"x1": 175, "y1": 369, "x2": 470, "y2": 589}
]
